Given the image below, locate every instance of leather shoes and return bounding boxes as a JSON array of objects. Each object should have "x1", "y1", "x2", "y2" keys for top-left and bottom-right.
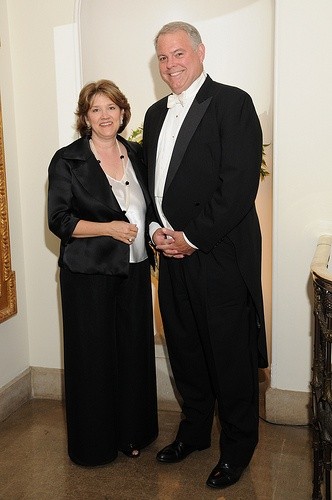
[
  {"x1": 155, "y1": 439, "x2": 211, "y2": 464},
  {"x1": 206, "y1": 460, "x2": 241, "y2": 488}
]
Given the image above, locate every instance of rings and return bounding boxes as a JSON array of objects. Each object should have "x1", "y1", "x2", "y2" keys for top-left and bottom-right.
[{"x1": 128, "y1": 238, "x2": 133, "y2": 244}]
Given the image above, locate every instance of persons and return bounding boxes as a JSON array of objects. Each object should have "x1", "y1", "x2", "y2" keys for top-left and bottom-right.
[
  {"x1": 47, "y1": 78, "x2": 161, "y2": 468},
  {"x1": 143, "y1": 21, "x2": 269, "y2": 491}
]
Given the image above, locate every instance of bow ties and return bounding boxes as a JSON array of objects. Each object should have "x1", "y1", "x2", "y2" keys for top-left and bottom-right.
[{"x1": 167, "y1": 91, "x2": 187, "y2": 108}]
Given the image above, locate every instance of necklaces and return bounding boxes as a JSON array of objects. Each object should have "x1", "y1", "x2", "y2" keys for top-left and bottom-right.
[{"x1": 90, "y1": 137, "x2": 129, "y2": 213}]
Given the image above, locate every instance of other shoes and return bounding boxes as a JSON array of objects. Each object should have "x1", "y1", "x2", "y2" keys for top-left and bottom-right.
[{"x1": 123, "y1": 441, "x2": 140, "y2": 458}]
